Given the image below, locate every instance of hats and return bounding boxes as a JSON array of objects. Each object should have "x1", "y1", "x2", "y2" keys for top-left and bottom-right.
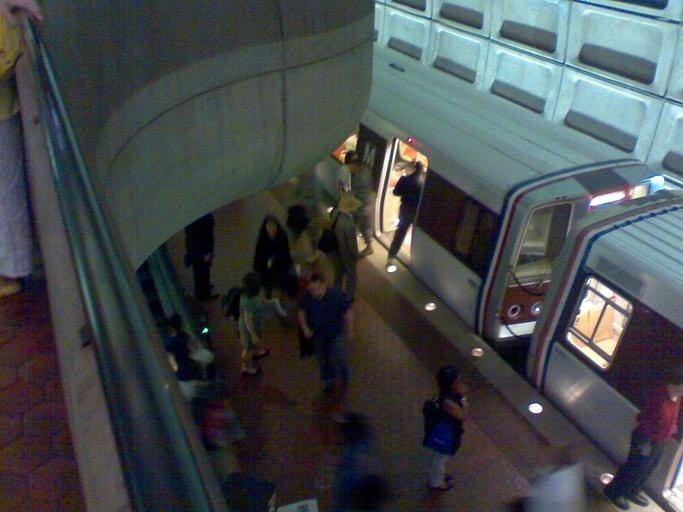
[{"x1": 337, "y1": 196, "x2": 362, "y2": 213}]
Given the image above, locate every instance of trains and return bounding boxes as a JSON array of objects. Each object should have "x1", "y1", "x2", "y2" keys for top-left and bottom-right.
[{"x1": 311, "y1": 43, "x2": 683, "y2": 512}]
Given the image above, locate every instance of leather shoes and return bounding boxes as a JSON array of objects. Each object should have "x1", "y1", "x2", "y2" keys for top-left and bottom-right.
[{"x1": 604, "y1": 487, "x2": 649, "y2": 510}]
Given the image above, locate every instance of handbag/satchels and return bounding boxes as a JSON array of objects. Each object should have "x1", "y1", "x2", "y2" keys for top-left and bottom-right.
[
  {"x1": 297, "y1": 324, "x2": 317, "y2": 361},
  {"x1": 317, "y1": 230, "x2": 338, "y2": 254},
  {"x1": 219, "y1": 286, "x2": 245, "y2": 322},
  {"x1": 422, "y1": 395, "x2": 461, "y2": 456}
]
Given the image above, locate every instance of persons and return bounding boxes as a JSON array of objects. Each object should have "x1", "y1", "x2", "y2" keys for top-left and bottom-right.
[
  {"x1": 385, "y1": 161, "x2": 423, "y2": 265},
  {"x1": 600, "y1": 370, "x2": 682, "y2": 511},
  {"x1": 0, "y1": 0, "x2": 44, "y2": 300},
  {"x1": 160, "y1": 152, "x2": 398, "y2": 511},
  {"x1": 427, "y1": 364, "x2": 468, "y2": 491},
  {"x1": 510, "y1": 435, "x2": 588, "y2": 511}
]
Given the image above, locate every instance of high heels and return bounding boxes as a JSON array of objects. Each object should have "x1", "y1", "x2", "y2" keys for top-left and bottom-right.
[
  {"x1": 428, "y1": 472, "x2": 453, "y2": 491},
  {"x1": 241, "y1": 349, "x2": 270, "y2": 377}
]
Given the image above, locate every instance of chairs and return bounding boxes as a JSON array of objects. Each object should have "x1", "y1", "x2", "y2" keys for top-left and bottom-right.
[{"x1": 571, "y1": 304, "x2": 622, "y2": 367}]
